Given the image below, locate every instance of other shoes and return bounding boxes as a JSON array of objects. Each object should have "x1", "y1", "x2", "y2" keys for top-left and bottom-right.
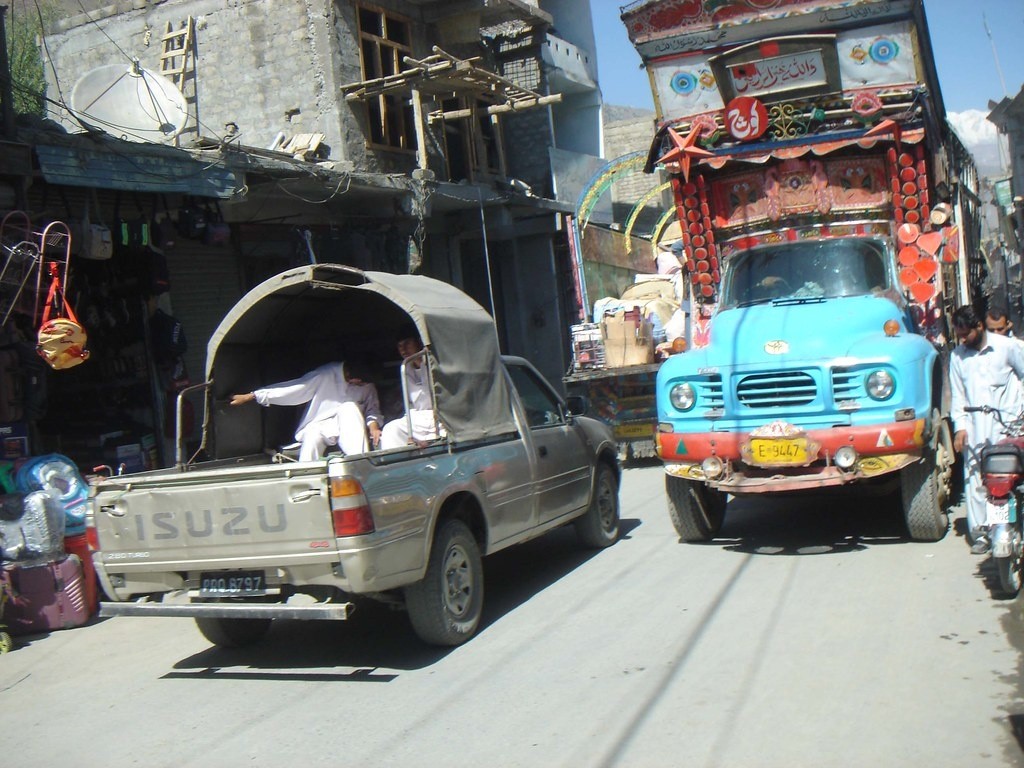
[{"x1": 971, "y1": 542, "x2": 990, "y2": 554}]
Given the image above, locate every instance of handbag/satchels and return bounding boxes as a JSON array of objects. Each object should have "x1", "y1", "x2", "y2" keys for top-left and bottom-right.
[
  {"x1": 148, "y1": 307, "x2": 187, "y2": 357},
  {"x1": 138, "y1": 253, "x2": 175, "y2": 292},
  {"x1": 157, "y1": 355, "x2": 190, "y2": 391},
  {"x1": 163, "y1": 395, "x2": 194, "y2": 437},
  {"x1": 36, "y1": 262, "x2": 89, "y2": 370},
  {"x1": 36, "y1": 191, "x2": 231, "y2": 258}
]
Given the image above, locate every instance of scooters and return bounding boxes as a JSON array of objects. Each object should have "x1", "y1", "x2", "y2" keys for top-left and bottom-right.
[{"x1": 961, "y1": 406, "x2": 1023, "y2": 595}]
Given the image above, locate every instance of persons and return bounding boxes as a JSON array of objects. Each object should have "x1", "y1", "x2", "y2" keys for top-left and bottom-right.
[
  {"x1": 983, "y1": 307, "x2": 1024, "y2": 349},
  {"x1": 949, "y1": 305, "x2": 1024, "y2": 554},
  {"x1": 381, "y1": 328, "x2": 447, "y2": 451},
  {"x1": 226, "y1": 353, "x2": 386, "y2": 462}
]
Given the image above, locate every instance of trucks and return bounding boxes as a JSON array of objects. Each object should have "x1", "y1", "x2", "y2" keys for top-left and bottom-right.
[{"x1": 626, "y1": 0, "x2": 993, "y2": 544}]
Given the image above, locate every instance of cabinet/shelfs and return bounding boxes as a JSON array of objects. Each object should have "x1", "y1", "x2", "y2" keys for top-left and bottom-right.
[{"x1": 0, "y1": 209, "x2": 71, "y2": 326}]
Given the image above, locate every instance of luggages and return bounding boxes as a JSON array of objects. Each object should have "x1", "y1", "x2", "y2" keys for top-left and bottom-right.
[
  {"x1": 0, "y1": 493, "x2": 63, "y2": 561},
  {"x1": 1, "y1": 554, "x2": 88, "y2": 637},
  {"x1": 0, "y1": 349, "x2": 23, "y2": 423}
]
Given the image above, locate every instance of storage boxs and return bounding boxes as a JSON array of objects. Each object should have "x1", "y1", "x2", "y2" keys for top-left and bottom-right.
[{"x1": 604, "y1": 310, "x2": 654, "y2": 369}]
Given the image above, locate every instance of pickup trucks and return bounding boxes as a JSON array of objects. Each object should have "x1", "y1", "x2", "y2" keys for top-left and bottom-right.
[{"x1": 82, "y1": 354, "x2": 622, "y2": 656}]
